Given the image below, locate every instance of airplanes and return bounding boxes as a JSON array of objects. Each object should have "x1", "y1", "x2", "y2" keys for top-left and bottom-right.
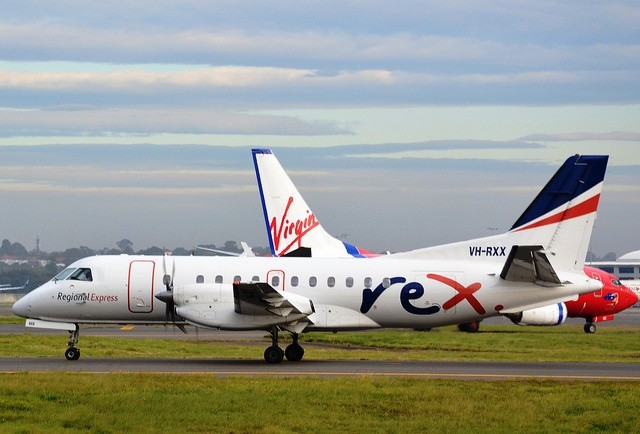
[{"x1": 11, "y1": 148, "x2": 639, "y2": 363}]
